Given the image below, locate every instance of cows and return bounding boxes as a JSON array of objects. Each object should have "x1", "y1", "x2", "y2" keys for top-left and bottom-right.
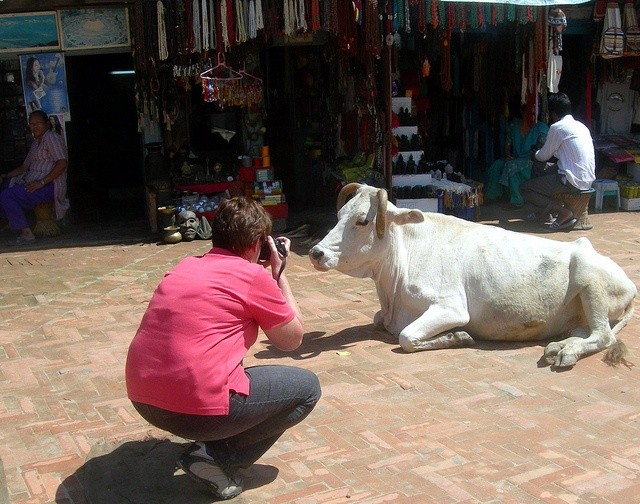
[{"x1": 308, "y1": 182, "x2": 638, "y2": 369}]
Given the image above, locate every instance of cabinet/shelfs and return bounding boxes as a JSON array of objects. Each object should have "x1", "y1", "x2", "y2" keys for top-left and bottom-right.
[
  {"x1": 392, "y1": 96, "x2": 439, "y2": 213},
  {"x1": 173, "y1": 180, "x2": 244, "y2": 221}
]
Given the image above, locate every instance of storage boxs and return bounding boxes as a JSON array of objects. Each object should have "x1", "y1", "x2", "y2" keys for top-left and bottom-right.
[
  {"x1": 265, "y1": 195, "x2": 285, "y2": 203},
  {"x1": 444, "y1": 181, "x2": 484, "y2": 208},
  {"x1": 621, "y1": 196, "x2": 640, "y2": 212},
  {"x1": 257, "y1": 168, "x2": 274, "y2": 182}
]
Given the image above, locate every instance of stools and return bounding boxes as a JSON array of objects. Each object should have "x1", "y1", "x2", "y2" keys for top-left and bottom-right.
[
  {"x1": 594, "y1": 191, "x2": 620, "y2": 211},
  {"x1": 144, "y1": 179, "x2": 172, "y2": 233},
  {"x1": 559, "y1": 188, "x2": 596, "y2": 230},
  {"x1": 33, "y1": 204, "x2": 60, "y2": 236},
  {"x1": 592, "y1": 179, "x2": 621, "y2": 212}
]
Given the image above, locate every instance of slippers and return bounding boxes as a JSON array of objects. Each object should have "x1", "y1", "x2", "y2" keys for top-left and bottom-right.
[
  {"x1": 547, "y1": 218, "x2": 577, "y2": 230},
  {"x1": 7, "y1": 238, "x2": 38, "y2": 245}
]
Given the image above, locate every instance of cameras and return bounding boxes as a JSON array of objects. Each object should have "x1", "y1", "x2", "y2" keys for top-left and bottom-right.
[{"x1": 258, "y1": 238, "x2": 287, "y2": 260}]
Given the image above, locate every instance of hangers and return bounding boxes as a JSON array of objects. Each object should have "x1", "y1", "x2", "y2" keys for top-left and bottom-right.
[
  {"x1": 201, "y1": 52, "x2": 243, "y2": 83},
  {"x1": 222, "y1": 62, "x2": 263, "y2": 87}
]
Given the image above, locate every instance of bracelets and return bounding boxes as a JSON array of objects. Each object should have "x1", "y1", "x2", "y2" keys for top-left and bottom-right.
[{"x1": 40, "y1": 178, "x2": 46, "y2": 185}]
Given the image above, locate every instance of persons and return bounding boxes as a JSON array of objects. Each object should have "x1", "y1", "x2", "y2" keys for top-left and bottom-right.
[
  {"x1": 0, "y1": 109, "x2": 68, "y2": 246},
  {"x1": 125, "y1": 196, "x2": 321, "y2": 501},
  {"x1": 48, "y1": 114, "x2": 63, "y2": 134},
  {"x1": 518, "y1": 91, "x2": 596, "y2": 233},
  {"x1": 407, "y1": 153, "x2": 417, "y2": 174},
  {"x1": 398, "y1": 106, "x2": 404, "y2": 125},
  {"x1": 26, "y1": 57, "x2": 48, "y2": 90},
  {"x1": 396, "y1": 152, "x2": 407, "y2": 174},
  {"x1": 417, "y1": 152, "x2": 428, "y2": 173},
  {"x1": 404, "y1": 106, "x2": 412, "y2": 124},
  {"x1": 485, "y1": 95, "x2": 549, "y2": 209},
  {"x1": 395, "y1": 133, "x2": 425, "y2": 150}
]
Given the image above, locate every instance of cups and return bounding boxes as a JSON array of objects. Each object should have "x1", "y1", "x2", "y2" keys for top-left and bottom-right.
[
  {"x1": 261, "y1": 146, "x2": 269, "y2": 156},
  {"x1": 262, "y1": 156, "x2": 271, "y2": 167},
  {"x1": 240, "y1": 156, "x2": 252, "y2": 168},
  {"x1": 252, "y1": 157, "x2": 262, "y2": 168}
]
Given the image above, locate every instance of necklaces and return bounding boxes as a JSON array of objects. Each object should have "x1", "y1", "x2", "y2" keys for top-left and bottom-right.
[{"x1": 126, "y1": 0, "x2": 539, "y2": 68}]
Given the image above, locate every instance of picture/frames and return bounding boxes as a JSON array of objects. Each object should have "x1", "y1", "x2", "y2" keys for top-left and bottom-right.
[
  {"x1": 56, "y1": 4, "x2": 132, "y2": 51},
  {"x1": 46, "y1": 113, "x2": 68, "y2": 146},
  {"x1": 0, "y1": 10, "x2": 61, "y2": 53}
]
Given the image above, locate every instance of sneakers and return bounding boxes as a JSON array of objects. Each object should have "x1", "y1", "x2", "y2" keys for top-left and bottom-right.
[{"x1": 177, "y1": 443, "x2": 243, "y2": 499}]
